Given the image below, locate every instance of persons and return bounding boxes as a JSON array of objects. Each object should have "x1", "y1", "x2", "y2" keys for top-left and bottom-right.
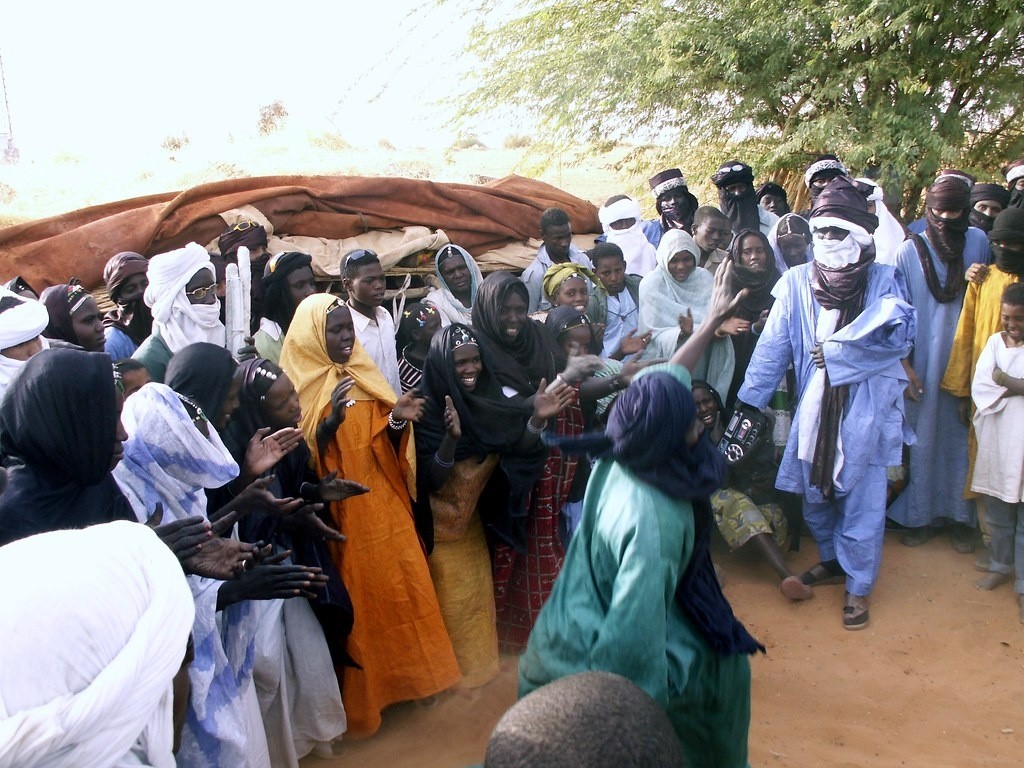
[{"x1": 0, "y1": 154, "x2": 1024, "y2": 768}]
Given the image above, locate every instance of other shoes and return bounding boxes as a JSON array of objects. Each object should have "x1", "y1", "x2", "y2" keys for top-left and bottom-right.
[
  {"x1": 780, "y1": 577, "x2": 814, "y2": 601},
  {"x1": 309, "y1": 741, "x2": 347, "y2": 760}
]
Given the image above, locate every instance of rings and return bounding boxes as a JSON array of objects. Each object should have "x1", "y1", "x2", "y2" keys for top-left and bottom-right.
[
  {"x1": 643, "y1": 339, "x2": 645, "y2": 342},
  {"x1": 737, "y1": 328, "x2": 743, "y2": 332},
  {"x1": 242, "y1": 560, "x2": 246, "y2": 568}
]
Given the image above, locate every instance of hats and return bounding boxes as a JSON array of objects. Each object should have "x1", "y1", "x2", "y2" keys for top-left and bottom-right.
[
  {"x1": 804, "y1": 155, "x2": 847, "y2": 189},
  {"x1": 1001, "y1": 158, "x2": 1024, "y2": 184},
  {"x1": 648, "y1": 168, "x2": 688, "y2": 201},
  {"x1": 934, "y1": 169, "x2": 977, "y2": 188}
]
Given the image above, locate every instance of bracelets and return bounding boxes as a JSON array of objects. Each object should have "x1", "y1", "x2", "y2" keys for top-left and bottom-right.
[
  {"x1": 527, "y1": 416, "x2": 548, "y2": 435},
  {"x1": 388, "y1": 412, "x2": 407, "y2": 430},
  {"x1": 750, "y1": 324, "x2": 760, "y2": 337}
]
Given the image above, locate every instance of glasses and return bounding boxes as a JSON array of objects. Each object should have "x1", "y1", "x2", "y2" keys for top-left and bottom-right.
[
  {"x1": 270, "y1": 250, "x2": 295, "y2": 273},
  {"x1": 719, "y1": 164, "x2": 746, "y2": 173},
  {"x1": 345, "y1": 249, "x2": 378, "y2": 267},
  {"x1": 186, "y1": 284, "x2": 219, "y2": 300},
  {"x1": 223, "y1": 220, "x2": 262, "y2": 235}
]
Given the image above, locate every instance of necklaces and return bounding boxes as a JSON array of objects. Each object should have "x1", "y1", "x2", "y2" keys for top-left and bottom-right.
[{"x1": 402, "y1": 342, "x2": 422, "y2": 373}]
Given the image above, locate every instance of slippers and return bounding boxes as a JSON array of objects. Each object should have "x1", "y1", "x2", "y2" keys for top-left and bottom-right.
[
  {"x1": 803, "y1": 562, "x2": 846, "y2": 587},
  {"x1": 842, "y1": 591, "x2": 869, "y2": 631}
]
